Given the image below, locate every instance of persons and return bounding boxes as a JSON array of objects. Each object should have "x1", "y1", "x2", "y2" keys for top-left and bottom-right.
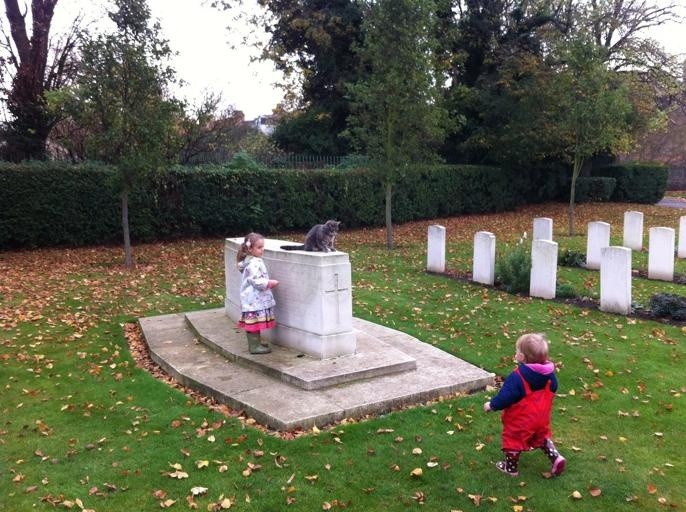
[
  {"x1": 483, "y1": 333, "x2": 567, "y2": 477},
  {"x1": 236, "y1": 232, "x2": 282, "y2": 354}
]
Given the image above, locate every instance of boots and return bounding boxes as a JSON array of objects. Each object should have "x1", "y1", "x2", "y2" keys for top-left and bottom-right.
[
  {"x1": 542, "y1": 440, "x2": 566, "y2": 477},
  {"x1": 247, "y1": 332, "x2": 270, "y2": 354},
  {"x1": 496, "y1": 450, "x2": 521, "y2": 477}
]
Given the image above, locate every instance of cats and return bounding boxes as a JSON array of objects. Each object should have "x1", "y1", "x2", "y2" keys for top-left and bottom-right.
[{"x1": 280, "y1": 219, "x2": 341, "y2": 253}]
